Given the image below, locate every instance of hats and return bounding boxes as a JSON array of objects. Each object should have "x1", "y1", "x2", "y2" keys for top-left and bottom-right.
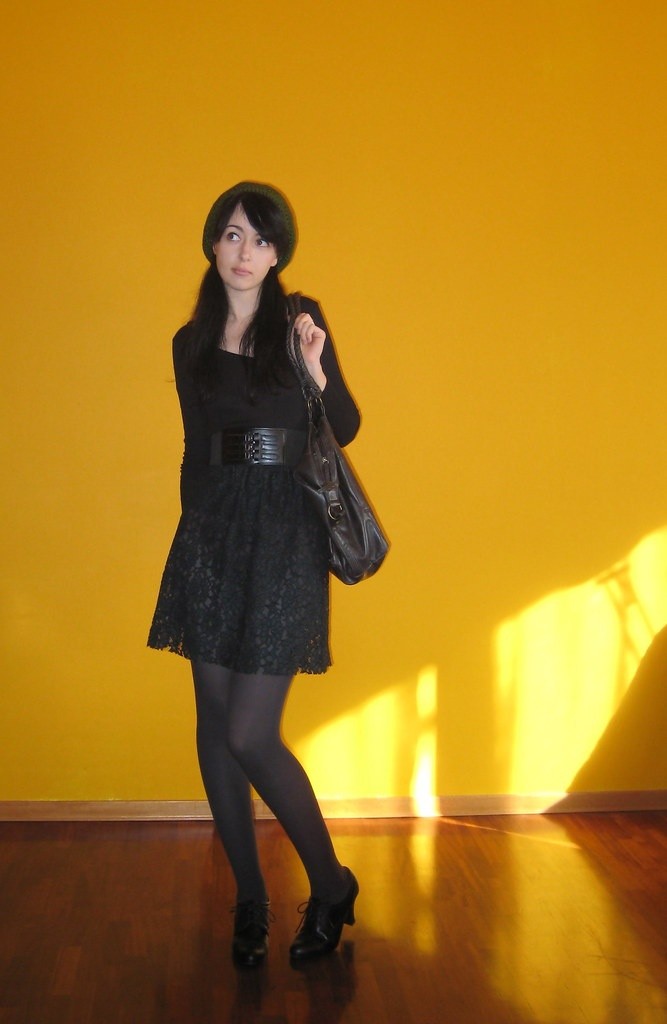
[{"x1": 202, "y1": 181, "x2": 298, "y2": 275}]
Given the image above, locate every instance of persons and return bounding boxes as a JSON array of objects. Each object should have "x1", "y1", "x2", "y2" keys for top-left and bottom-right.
[{"x1": 148, "y1": 182, "x2": 361, "y2": 984}]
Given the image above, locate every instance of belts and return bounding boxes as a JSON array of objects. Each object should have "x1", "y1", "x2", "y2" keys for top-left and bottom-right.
[{"x1": 189, "y1": 427, "x2": 305, "y2": 466}]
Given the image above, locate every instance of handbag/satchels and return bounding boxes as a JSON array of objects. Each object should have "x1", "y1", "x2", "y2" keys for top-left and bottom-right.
[{"x1": 286, "y1": 291, "x2": 390, "y2": 586}]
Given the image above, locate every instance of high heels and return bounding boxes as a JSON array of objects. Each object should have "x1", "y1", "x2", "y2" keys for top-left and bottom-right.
[
  {"x1": 228, "y1": 893, "x2": 276, "y2": 969},
  {"x1": 289, "y1": 866, "x2": 359, "y2": 961}
]
